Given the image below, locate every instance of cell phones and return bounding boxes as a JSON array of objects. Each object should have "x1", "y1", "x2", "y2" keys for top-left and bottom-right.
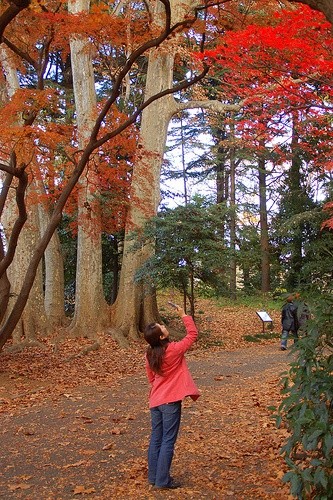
[{"x1": 168, "y1": 302, "x2": 177, "y2": 308}]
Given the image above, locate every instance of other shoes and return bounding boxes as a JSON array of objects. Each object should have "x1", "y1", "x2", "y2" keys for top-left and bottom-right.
[
  {"x1": 281, "y1": 347, "x2": 286, "y2": 350},
  {"x1": 153, "y1": 477, "x2": 181, "y2": 489}
]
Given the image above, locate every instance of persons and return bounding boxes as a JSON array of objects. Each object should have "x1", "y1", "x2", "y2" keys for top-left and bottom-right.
[
  {"x1": 143, "y1": 304, "x2": 200, "y2": 489},
  {"x1": 280, "y1": 292, "x2": 311, "y2": 350}
]
